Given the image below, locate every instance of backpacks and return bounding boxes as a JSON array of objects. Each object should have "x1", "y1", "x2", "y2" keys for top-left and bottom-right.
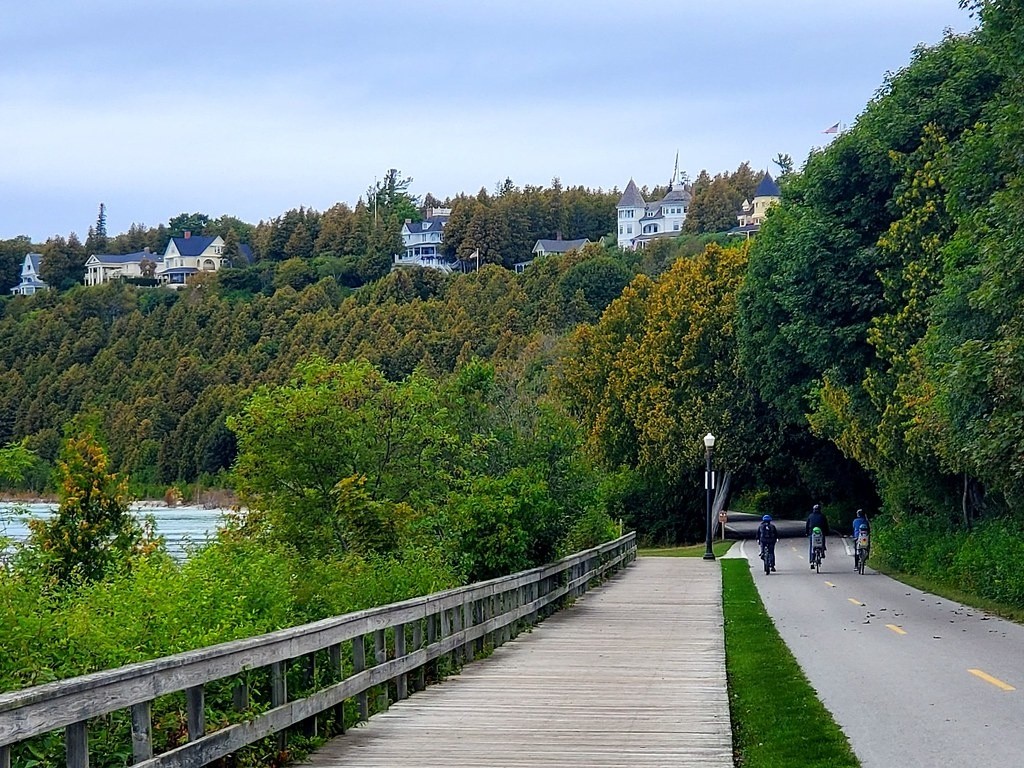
[{"x1": 760, "y1": 523, "x2": 774, "y2": 544}]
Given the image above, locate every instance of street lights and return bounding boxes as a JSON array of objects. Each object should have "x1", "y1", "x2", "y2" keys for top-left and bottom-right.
[{"x1": 703, "y1": 433, "x2": 715, "y2": 560}]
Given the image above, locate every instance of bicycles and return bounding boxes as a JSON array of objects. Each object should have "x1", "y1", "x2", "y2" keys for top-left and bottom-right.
[
  {"x1": 852, "y1": 535, "x2": 871, "y2": 576},
  {"x1": 806, "y1": 532, "x2": 825, "y2": 574},
  {"x1": 758, "y1": 538, "x2": 777, "y2": 575}
]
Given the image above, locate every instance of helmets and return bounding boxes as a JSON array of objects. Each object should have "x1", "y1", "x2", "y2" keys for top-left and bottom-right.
[
  {"x1": 813, "y1": 504, "x2": 821, "y2": 510},
  {"x1": 813, "y1": 527, "x2": 821, "y2": 534},
  {"x1": 763, "y1": 515, "x2": 773, "y2": 521},
  {"x1": 859, "y1": 524, "x2": 867, "y2": 533}
]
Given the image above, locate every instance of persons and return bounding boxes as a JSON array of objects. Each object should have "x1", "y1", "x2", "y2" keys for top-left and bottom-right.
[
  {"x1": 853, "y1": 508, "x2": 871, "y2": 571},
  {"x1": 756, "y1": 515, "x2": 779, "y2": 572},
  {"x1": 806, "y1": 504, "x2": 829, "y2": 569}
]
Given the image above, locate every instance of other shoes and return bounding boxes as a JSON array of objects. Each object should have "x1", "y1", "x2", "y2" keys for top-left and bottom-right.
[
  {"x1": 811, "y1": 564, "x2": 815, "y2": 569},
  {"x1": 759, "y1": 550, "x2": 764, "y2": 556},
  {"x1": 853, "y1": 567, "x2": 858, "y2": 571},
  {"x1": 771, "y1": 568, "x2": 776, "y2": 572},
  {"x1": 821, "y1": 551, "x2": 825, "y2": 558}
]
